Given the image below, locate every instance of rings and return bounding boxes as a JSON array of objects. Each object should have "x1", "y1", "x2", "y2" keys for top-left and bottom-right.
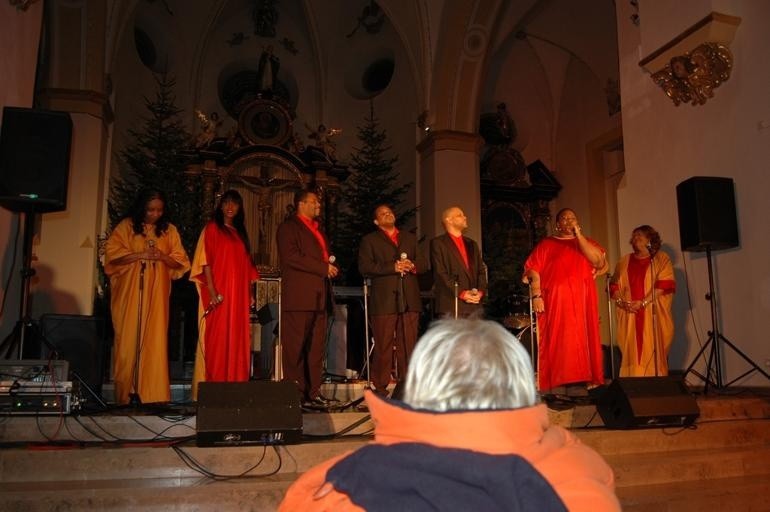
[
  {"x1": 154, "y1": 253, "x2": 158, "y2": 256},
  {"x1": 209, "y1": 301, "x2": 213, "y2": 305}
]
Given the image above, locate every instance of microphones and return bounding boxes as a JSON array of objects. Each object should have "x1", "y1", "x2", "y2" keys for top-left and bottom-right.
[
  {"x1": 148, "y1": 240, "x2": 156, "y2": 264},
  {"x1": 328, "y1": 255, "x2": 336, "y2": 277},
  {"x1": 400, "y1": 253, "x2": 407, "y2": 277},
  {"x1": 645, "y1": 243, "x2": 655, "y2": 254},
  {"x1": 203, "y1": 294, "x2": 223, "y2": 314},
  {"x1": 572, "y1": 226, "x2": 576, "y2": 238},
  {"x1": 472, "y1": 288, "x2": 478, "y2": 296}
]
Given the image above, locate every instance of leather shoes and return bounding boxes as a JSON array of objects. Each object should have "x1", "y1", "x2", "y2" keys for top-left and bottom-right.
[
  {"x1": 315, "y1": 395, "x2": 340, "y2": 404},
  {"x1": 302, "y1": 401, "x2": 321, "y2": 414}
]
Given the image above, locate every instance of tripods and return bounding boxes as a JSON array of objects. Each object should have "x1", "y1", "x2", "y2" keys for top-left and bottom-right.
[
  {"x1": 337, "y1": 279, "x2": 375, "y2": 412},
  {"x1": 528, "y1": 278, "x2": 578, "y2": 403},
  {"x1": 0, "y1": 211, "x2": 108, "y2": 408},
  {"x1": 684, "y1": 252, "x2": 769, "y2": 395}
]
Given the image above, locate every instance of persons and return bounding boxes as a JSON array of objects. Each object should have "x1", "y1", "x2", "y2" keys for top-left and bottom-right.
[
  {"x1": 236, "y1": 172, "x2": 297, "y2": 240},
  {"x1": 356, "y1": 204, "x2": 430, "y2": 404},
  {"x1": 667, "y1": 55, "x2": 693, "y2": 80},
  {"x1": 603, "y1": 224, "x2": 677, "y2": 378},
  {"x1": 304, "y1": 119, "x2": 334, "y2": 159},
  {"x1": 195, "y1": 109, "x2": 227, "y2": 148},
  {"x1": 187, "y1": 188, "x2": 262, "y2": 404},
  {"x1": 520, "y1": 207, "x2": 610, "y2": 401},
  {"x1": 428, "y1": 206, "x2": 489, "y2": 320},
  {"x1": 274, "y1": 188, "x2": 343, "y2": 411},
  {"x1": 267, "y1": 314, "x2": 624, "y2": 510},
  {"x1": 102, "y1": 186, "x2": 193, "y2": 408},
  {"x1": 257, "y1": 43, "x2": 281, "y2": 92}
]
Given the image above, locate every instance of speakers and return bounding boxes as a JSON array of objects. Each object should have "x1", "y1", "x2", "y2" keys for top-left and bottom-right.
[
  {"x1": 39, "y1": 314, "x2": 114, "y2": 405},
  {"x1": 0, "y1": 106, "x2": 74, "y2": 215},
  {"x1": 195, "y1": 381, "x2": 304, "y2": 442},
  {"x1": 596, "y1": 376, "x2": 699, "y2": 429},
  {"x1": 675, "y1": 175, "x2": 740, "y2": 251}
]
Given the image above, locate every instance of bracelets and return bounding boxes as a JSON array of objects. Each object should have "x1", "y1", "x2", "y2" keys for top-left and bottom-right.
[
  {"x1": 641, "y1": 297, "x2": 648, "y2": 308},
  {"x1": 616, "y1": 296, "x2": 623, "y2": 306},
  {"x1": 573, "y1": 226, "x2": 581, "y2": 238},
  {"x1": 532, "y1": 295, "x2": 542, "y2": 299}
]
